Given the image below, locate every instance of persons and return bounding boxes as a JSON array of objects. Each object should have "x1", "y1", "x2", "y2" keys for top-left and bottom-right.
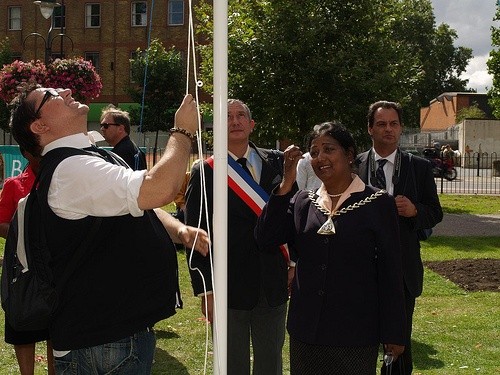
[
  {"x1": 253, "y1": 121, "x2": 407, "y2": 375},
  {"x1": 183, "y1": 98, "x2": 297, "y2": 375},
  {"x1": 0, "y1": 142, "x2": 55, "y2": 374},
  {"x1": 172, "y1": 157, "x2": 204, "y2": 249},
  {"x1": 350, "y1": 101, "x2": 443, "y2": 375},
  {"x1": 100, "y1": 103, "x2": 147, "y2": 171},
  {"x1": 8, "y1": 82, "x2": 202, "y2": 375},
  {"x1": 422, "y1": 141, "x2": 457, "y2": 180}
]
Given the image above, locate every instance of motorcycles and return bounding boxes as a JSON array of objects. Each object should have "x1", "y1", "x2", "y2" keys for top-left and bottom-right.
[{"x1": 434, "y1": 158, "x2": 458, "y2": 181}]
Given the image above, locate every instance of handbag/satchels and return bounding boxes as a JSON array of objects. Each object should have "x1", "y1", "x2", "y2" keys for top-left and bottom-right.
[{"x1": 401, "y1": 151, "x2": 433, "y2": 241}]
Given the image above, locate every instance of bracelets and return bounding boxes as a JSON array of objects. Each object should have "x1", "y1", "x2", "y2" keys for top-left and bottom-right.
[{"x1": 168, "y1": 127, "x2": 197, "y2": 149}]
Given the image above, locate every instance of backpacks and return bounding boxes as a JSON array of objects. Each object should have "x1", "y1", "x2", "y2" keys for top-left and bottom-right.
[{"x1": 0, "y1": 165, "x2": 54, "y2": 333}]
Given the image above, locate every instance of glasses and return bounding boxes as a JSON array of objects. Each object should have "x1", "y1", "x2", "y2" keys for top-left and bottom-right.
[
  {"x1": 101, "y1": 123, "x2": 120, "y2": 129},
  {"x1": 33, "y1": 88, "x2": 64, "y2": 122}
]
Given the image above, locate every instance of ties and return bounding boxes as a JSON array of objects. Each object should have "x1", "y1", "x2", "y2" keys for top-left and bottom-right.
[
  {"x1": 376, "y1": 159, "x2": 388, "y2": 189},
  {"x1": 236, "y1": 157, "x2": 253, "y2": 179}
]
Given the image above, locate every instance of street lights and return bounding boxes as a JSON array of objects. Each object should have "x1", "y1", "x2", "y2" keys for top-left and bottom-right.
[{"x1": 23, "y1": 0, "x2": 73, "y2": 66}]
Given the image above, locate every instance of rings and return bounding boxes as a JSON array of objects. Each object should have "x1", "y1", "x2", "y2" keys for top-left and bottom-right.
[{"x1": 288, "y1": 156, "x2": 294, "y2": 161}]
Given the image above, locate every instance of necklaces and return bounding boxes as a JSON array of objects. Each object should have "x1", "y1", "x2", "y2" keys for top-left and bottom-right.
[{"x1": 307, "y1": 188, "x2": 387, "y2": 234}]
